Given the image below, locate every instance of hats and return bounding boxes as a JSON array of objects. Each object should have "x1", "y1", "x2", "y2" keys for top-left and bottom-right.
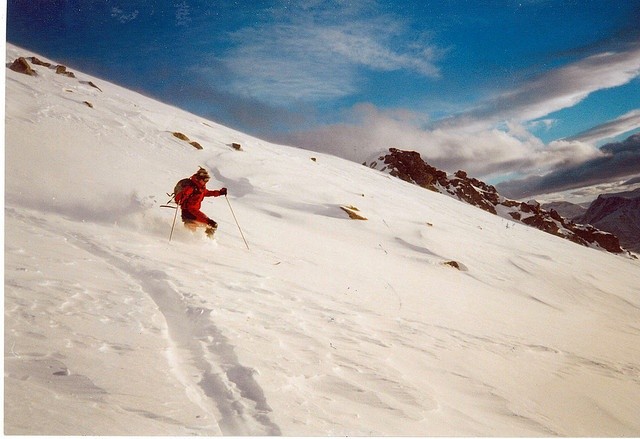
[{"x1": 197, "y1": 166, "x2": 209, "y2": 177}]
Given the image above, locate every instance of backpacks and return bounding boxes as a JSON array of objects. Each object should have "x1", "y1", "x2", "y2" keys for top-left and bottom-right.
[{"x1": 166, "y1": 178, "x2": 198, "y2": 204}]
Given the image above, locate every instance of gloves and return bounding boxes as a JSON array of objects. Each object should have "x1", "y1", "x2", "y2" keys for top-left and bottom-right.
[{"x1": 220, "y1": 188, "x2": 227, "y2": 195}]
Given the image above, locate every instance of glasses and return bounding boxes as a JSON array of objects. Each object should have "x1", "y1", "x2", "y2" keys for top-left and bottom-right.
[{"x1": 203, "y1": 178, "x2": 210, "y2": 182}]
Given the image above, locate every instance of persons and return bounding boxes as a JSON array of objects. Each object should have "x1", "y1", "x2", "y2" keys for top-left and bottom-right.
[{"x1": 175, "y1": 168, "x2": 227, "y2": 237}]
